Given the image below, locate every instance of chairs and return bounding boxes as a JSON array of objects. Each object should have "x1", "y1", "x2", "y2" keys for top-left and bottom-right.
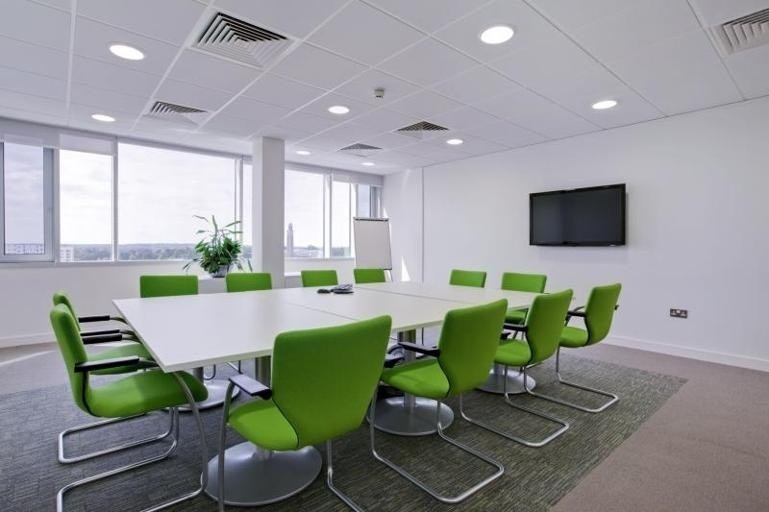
[
  {"x1": 519, "y1": 282, "x2": 622, "y2": 414},
  {"x1": 459, "y1": 288, "x2": 574, "y2": 449},
  {"x1": 450, "y1": 268, "x2": 488, "y2": 288},
  {"x1": 49, "y1": 302, "x2": 209, "y2": 511},
  {"x1": 369, "y1": 297, "x2": 510, "y2": 505},
  {"x1": 353, "y1": 268, "x2": 386, "y2": 283},
  {"x1": 139, "y1": 273, "x2": 246, "y2": 384},
  {"x1": 301, "y1": 269, "x2": 339, "y2": 287},
  {"x1": 219, "y1": 314, "x2": 396, "y2": 512},
  {"x1": 48, "y1": 290, "x2": 176, "y2": 465},
  {"x1": 225, "y1": 272, "x2": 273, "y2": 378},
  {"x1": 494, "y1": 271, "x2": 547, "y2": 372}
]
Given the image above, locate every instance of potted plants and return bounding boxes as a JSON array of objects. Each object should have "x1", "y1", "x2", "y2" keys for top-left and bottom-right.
[{"x1": 183, "y1": 214, "x2": 253, "y2": 279}]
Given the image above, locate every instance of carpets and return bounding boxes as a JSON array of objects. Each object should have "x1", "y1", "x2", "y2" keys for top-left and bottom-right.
[{"x1": 1, "y1": 335, "x2": 689, "y2": 512}]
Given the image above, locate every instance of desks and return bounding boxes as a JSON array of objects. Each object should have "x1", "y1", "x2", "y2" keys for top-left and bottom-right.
[{"x1": 111, "y1": 280, "x2": 555, "y2": 508}]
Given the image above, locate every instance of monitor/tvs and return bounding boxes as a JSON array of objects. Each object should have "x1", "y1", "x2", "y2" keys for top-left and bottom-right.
[{"x1": 529, "y1": 183, "x2": 626, "y2": 247}]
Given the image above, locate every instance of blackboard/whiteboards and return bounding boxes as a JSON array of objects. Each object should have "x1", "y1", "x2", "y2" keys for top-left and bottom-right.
[{"x1": 352, "y1": 217, "x2": 394, "y2": 270}]
